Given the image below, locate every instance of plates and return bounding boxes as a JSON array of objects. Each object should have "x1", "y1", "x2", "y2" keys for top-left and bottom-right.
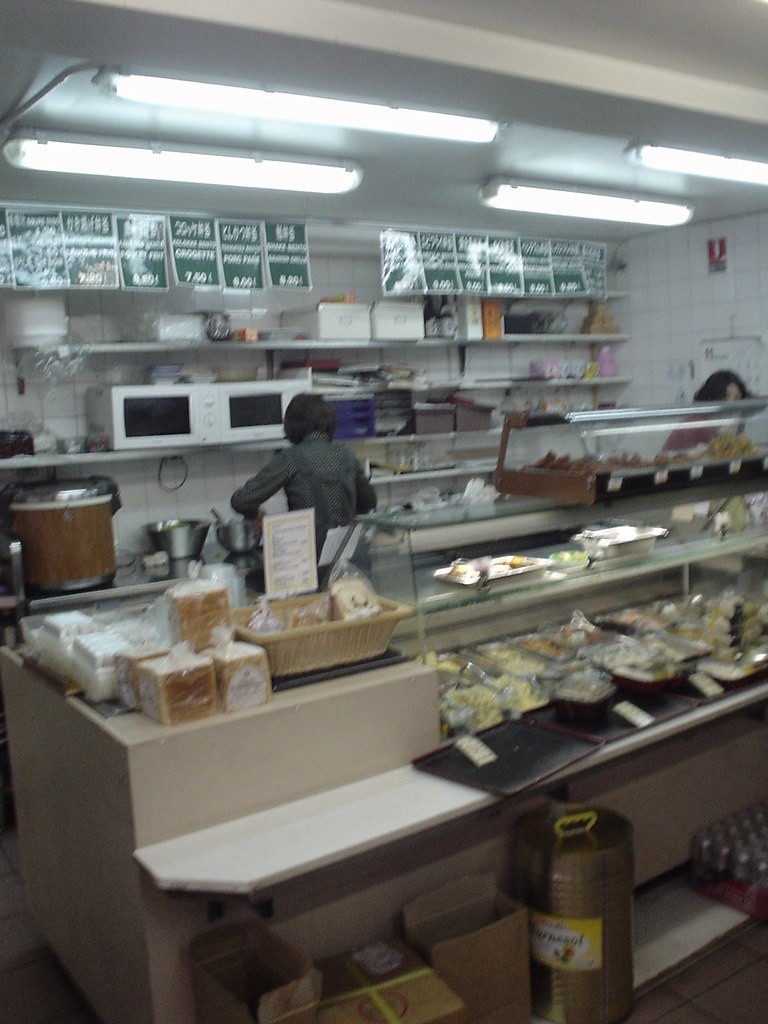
[
  {"x1": 6, "y1": 300, "x2": 67, "y2": 347},
  {"x1": 143, "y1": 363, "x2": 217, "y2": 383}
]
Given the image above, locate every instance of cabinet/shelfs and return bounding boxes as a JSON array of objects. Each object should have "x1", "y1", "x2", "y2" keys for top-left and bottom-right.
[
  {"x1": 0, "y1": 288, "x2": 631, "y2": 484},
  {"x1": 0, "y1": 647, "x2": 440, "y2": 1024}
]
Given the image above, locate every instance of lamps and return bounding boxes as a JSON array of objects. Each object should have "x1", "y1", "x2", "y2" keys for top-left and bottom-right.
[{"x1": 0, "y1": 59, "x2": 768, "y2": 227}]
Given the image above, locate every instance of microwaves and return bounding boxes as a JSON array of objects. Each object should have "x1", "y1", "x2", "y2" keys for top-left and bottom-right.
[
  {"x1": 86, "y1": 383, "x2": 217, "y2": 448},
  {"x1": 217, "y1": 382, "x2": 308, "y2": 446}
]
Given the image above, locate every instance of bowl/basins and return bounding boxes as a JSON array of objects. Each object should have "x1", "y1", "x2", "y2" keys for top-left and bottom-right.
[
  {"x1": 552, "y1": 681, "x2": 617, "y2": 723},
  {"x1": 146, "y1": 517, "x2": 210, "y2": 558},
  {"x1": 214, "y1": 519, "x2": 261, "y2": 554}
]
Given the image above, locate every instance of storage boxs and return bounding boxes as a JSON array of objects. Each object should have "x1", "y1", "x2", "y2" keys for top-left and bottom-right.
[
  {"x1": 180, "y1": 870, "x2": 533, "y2": 1024},
  {"x1": 372, "y1": 301, "x2": 425, "y2": 342},
  {"x1": 151, "y1": 312, "x2": 207, "y2": 341},
  {"x1": 317, "y1": 302, "x2": 371, "y2": 340}
]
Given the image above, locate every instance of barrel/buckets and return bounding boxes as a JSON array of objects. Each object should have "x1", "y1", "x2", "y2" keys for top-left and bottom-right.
[{"x1": 514, "y1": 802, "x2": 636, "y2": 1024}]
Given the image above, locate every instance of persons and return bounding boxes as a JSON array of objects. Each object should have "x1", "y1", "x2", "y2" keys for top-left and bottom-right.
[
  {"x1": 661, "y1": 370, "x2": 750, "y2": 453},
  {"x1": 230, "y1": 393, "x2": 378, "y2": 577}
]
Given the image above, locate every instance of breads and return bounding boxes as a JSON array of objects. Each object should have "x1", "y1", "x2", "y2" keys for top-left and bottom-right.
[
  {"x1": 112, "y1": 578, "x2": 272, "y2": 727},
  {"x1": 249, "y1": 574, "x2": 380, "y2": 633}
]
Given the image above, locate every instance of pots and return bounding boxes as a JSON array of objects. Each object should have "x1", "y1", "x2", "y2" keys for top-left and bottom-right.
[{"x1": 0, "y1": 475, "x2": 120, "y2": 593}]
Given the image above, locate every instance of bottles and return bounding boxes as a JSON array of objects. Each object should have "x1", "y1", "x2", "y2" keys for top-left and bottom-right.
[{"x1": 421, "y1": 294, "x2": 459, "y2": 339}]
